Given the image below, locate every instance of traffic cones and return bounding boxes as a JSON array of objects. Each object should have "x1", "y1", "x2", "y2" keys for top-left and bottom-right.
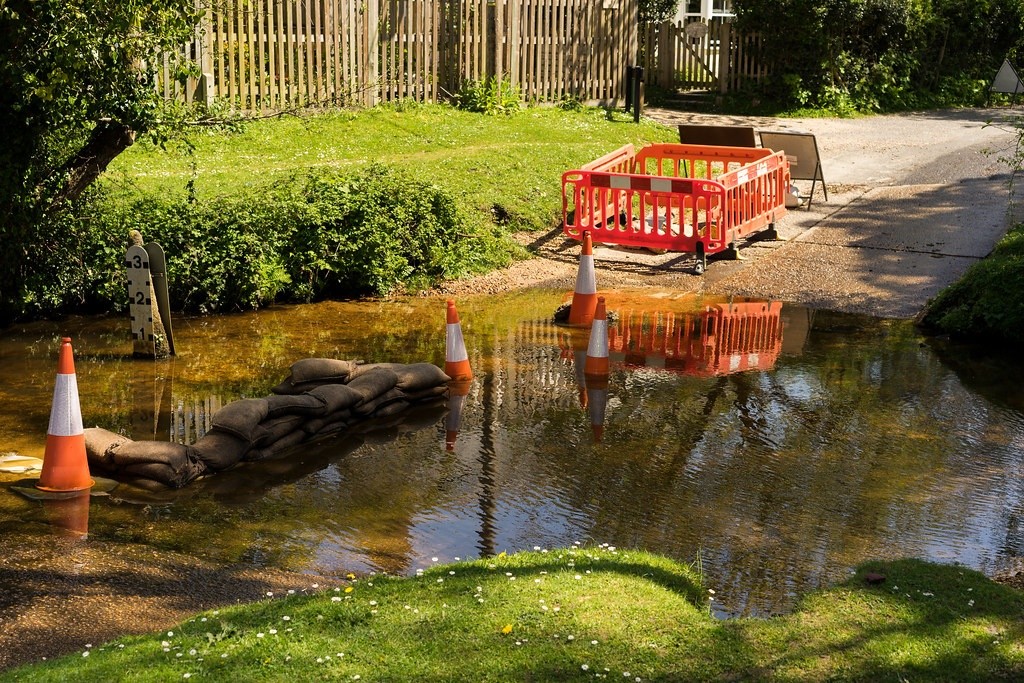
[
  {"x1": 571, "y1": 329, "x2": 597, "y2": 410},
  {"x1": 551, "y1": 231, "x2": 602, "y2": 329},
  {"x1": 10, "y1": 339, "x2": 110, "y2": 504},
  {"x1": 583, "y1": 297, "x2": 615, "y2": 379},
  {"x1": 586, "y1": 376, "x2": 619, "y2": 443},
  {"x1": 53, "y1": 490, "x2": 94, "y2": 536},
  {"x1": 434, "y1": 299, "x2": 478, "y2": 386},
  {"x1": 443, "y1": 386, "x2": 473, "y2": 456}
]
